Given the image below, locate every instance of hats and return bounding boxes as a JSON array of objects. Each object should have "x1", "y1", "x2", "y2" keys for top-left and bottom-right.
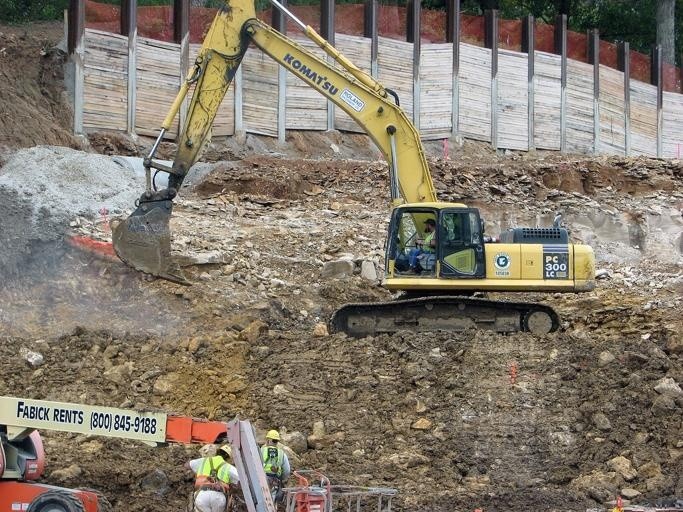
[{"x1": 424, "y1": 219, "x2": 436, "y2": 226}]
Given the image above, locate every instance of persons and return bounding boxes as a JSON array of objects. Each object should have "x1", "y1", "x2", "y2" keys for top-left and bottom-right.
[
  {"x1": 259, "y1": 430, "x2": 291, "y2": 503},
  {"x1": 401, "y1": 218, "x2": 437, "y2": 275},
  {"x1": 184, "y1": 444, "x2": 240, "y2": 512}
]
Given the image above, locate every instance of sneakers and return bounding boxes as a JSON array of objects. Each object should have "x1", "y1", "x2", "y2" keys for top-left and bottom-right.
[{"x1": 401, "y1": 267, "x2": 417, "y2": 275}]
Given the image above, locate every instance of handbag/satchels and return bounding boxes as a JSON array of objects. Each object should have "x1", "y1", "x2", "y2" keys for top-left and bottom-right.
[{"x1": 276, "y1": 481, "x2": 285, "y2": 501}]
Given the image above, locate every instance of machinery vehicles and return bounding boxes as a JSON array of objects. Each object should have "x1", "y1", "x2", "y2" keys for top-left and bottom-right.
[
  {"x1": 109, "y1": 1, "x2": 597, "y2": 338},
  {"x1": 1, "y1": 394, "x2": 279, "y2": 512}
]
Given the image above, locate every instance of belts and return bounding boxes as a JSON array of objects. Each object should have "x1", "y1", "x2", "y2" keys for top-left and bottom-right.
[
  {"x1": 201, "y1": 486, "x2": 224, "y2": 492},
  {"x1": 265, "y1": 472, "x2": 279, "y2": 478}
]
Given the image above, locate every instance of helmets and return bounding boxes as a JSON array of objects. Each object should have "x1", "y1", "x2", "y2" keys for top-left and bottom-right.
[
  {"x1": 266, "y1": 430, "x2": 281, "y2": 441},
  {"x1": 220, "y1": 445, "x2": 232, "y2": 459}
]
